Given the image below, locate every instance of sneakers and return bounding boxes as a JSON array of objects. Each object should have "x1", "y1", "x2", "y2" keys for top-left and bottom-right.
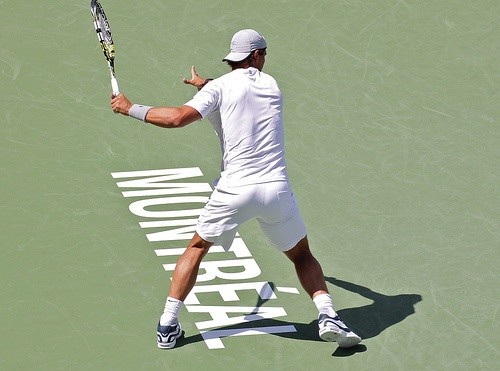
[
  {"x1": 156, "y1": 320, "x2": 183, "y2": 349},
  {"x1": 317, "y1": 312, "x2": 362, "y2": 348}
]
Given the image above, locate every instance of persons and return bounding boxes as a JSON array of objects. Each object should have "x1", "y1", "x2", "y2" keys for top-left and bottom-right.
[{"x1": 109, "y1": 28, "x2": 363, "y2": 351}]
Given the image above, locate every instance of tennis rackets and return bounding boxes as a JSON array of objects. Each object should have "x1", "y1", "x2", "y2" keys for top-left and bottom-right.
[{"x1": 90, "y1": 0, "x2": 121, "y2": 115}]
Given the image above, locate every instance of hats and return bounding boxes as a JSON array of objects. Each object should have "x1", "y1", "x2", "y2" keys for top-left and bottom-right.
[{"x1": 222, "y1": 28, "x2": 267, "y2": 63}]
